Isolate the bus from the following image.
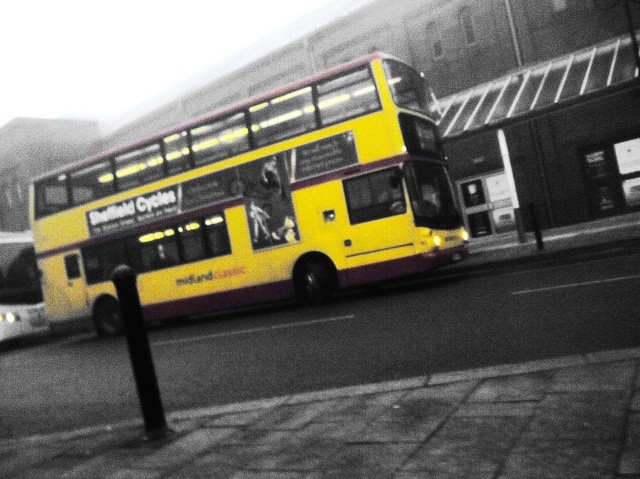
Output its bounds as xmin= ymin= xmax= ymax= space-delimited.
xmin=0 ymin=233 xmax=50 ymax=343
xmin=29 ymin=52 xmax=470 ymax=337
